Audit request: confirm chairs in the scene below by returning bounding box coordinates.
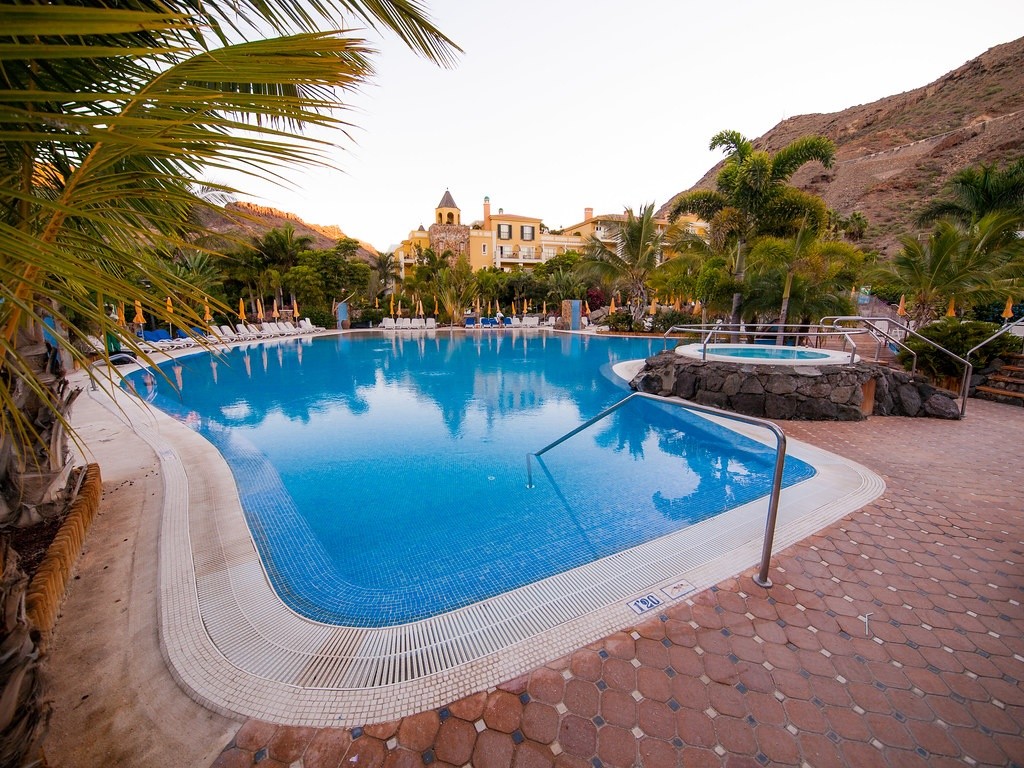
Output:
[220,325,244,343]
[209,326,231,344]
[176,329,198,348]
[236,324,257,341]
[873,321,919,338]
[276,322,296,337]
[401,318,410,329]
[299,320,316,333]
[306,318,327,333]
[384,319,395,329]
[284,322,307,335]
[136,331,170,351]
[247,324,270,340]
[269,323,287,338]
[378,318,389,328]
[393,318,403,329]
[260,322,281,339]
[410,317,588,330]
[192,327,217,346]
[152,329,184,350]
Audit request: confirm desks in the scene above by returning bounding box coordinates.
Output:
[892,330,905,337]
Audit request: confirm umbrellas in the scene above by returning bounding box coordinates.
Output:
[239,298,246,324]
[292,298,300,323]
[1001,293,1014,330]
[361,292,550,323]
[849,286,855,302]
[165,296,175,339]
[132,298,147,341]
[202,296,212,336]
[585,301,592,325]
[256,298,264,322]
[692,299,702,316]
[897,293,906,324]
[611,297,615,313]
[945,294,956,317]
[651,298,657,315]
[617,292,621,303]
[655,287,693,312]
[272,299,280,322]
[112,302,127,338]
[609,305,612,315]
